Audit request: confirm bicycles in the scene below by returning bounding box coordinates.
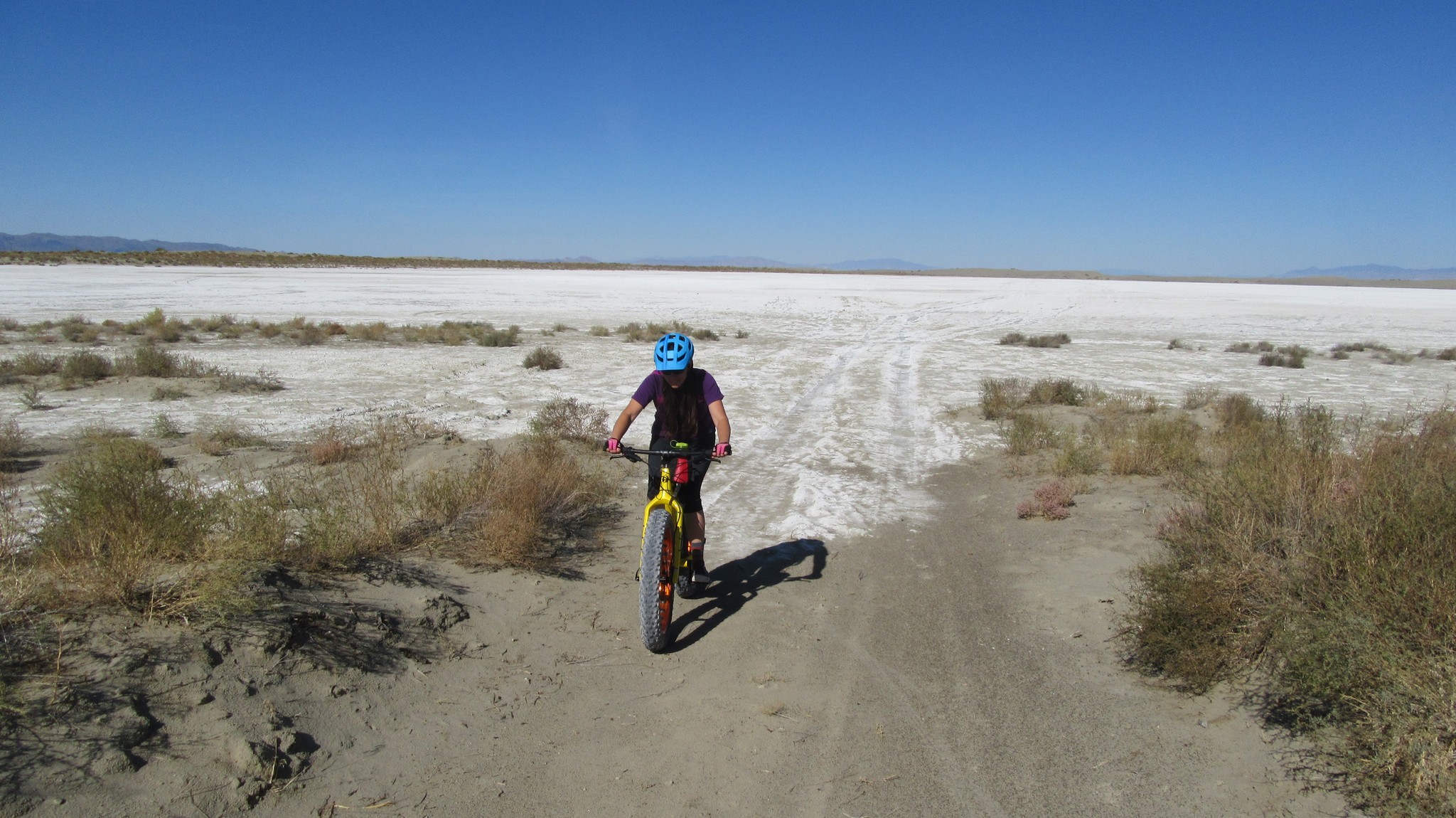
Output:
[602,444,733,654]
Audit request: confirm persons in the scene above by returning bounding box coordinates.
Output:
[603,334,730,584]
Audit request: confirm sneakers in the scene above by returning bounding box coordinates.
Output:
[687,548,710,583]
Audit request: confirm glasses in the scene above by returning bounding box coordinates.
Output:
[662,367,686,375]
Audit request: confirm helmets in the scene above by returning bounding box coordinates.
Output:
[654,333,694,370]
